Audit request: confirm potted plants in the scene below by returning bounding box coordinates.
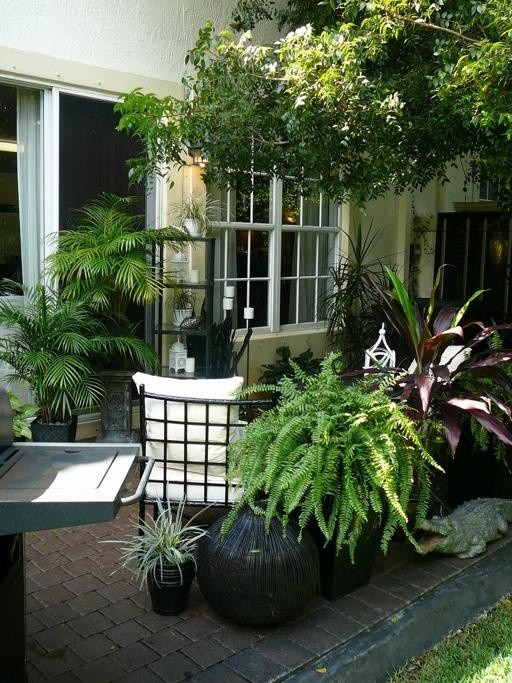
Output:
[44,189,197,443]
[164,275,201,328]
[167,184,223,238]
[0,277,160,442]
[342,261,512,540]
[218,342,446,603]
[97,488,217,615]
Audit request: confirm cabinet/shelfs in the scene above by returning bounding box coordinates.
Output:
[151,234,216,379]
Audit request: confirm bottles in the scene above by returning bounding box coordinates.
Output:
[169,334,188,377]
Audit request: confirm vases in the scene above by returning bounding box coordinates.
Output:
[452,202,479,211]
[489,232,508,273]
